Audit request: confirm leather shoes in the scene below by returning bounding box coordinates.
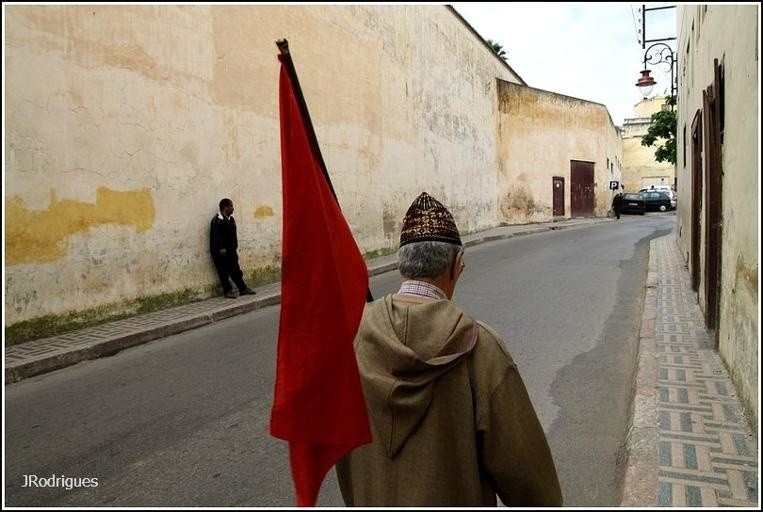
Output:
[240,288,256,295]
[224,292,237,299]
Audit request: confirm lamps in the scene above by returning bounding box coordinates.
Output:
[634,42,679,97]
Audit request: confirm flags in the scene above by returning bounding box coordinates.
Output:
[267,57,374,506]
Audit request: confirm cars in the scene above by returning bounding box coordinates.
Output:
[621,186,677,215]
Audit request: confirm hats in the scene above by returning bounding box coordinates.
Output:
[398,192,462,246]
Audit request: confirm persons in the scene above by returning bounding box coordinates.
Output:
[331,188,564,506]
[611,193,622,219]
[209,196,256,298]
